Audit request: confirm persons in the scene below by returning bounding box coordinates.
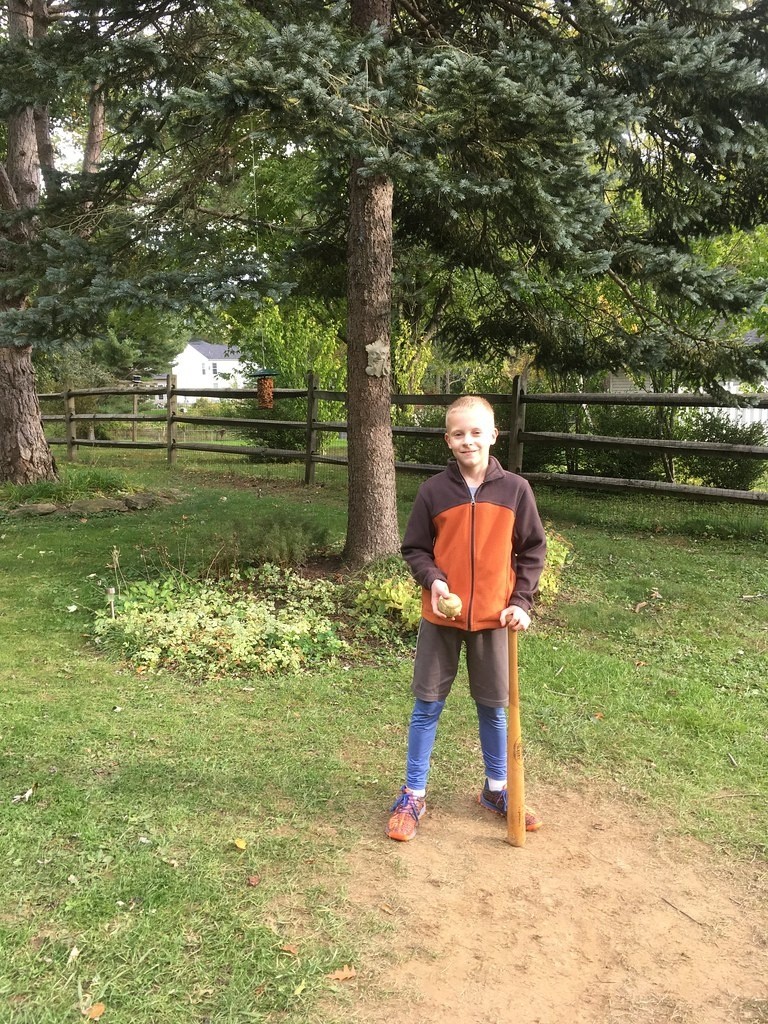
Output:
[387,395,549,842]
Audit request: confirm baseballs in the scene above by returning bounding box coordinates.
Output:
[437,592,462,618]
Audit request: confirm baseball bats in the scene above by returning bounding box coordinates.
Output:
[505,615,527,849]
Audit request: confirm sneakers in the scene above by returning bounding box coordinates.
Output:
[478,778,543,831]
[386,788,426,840]
